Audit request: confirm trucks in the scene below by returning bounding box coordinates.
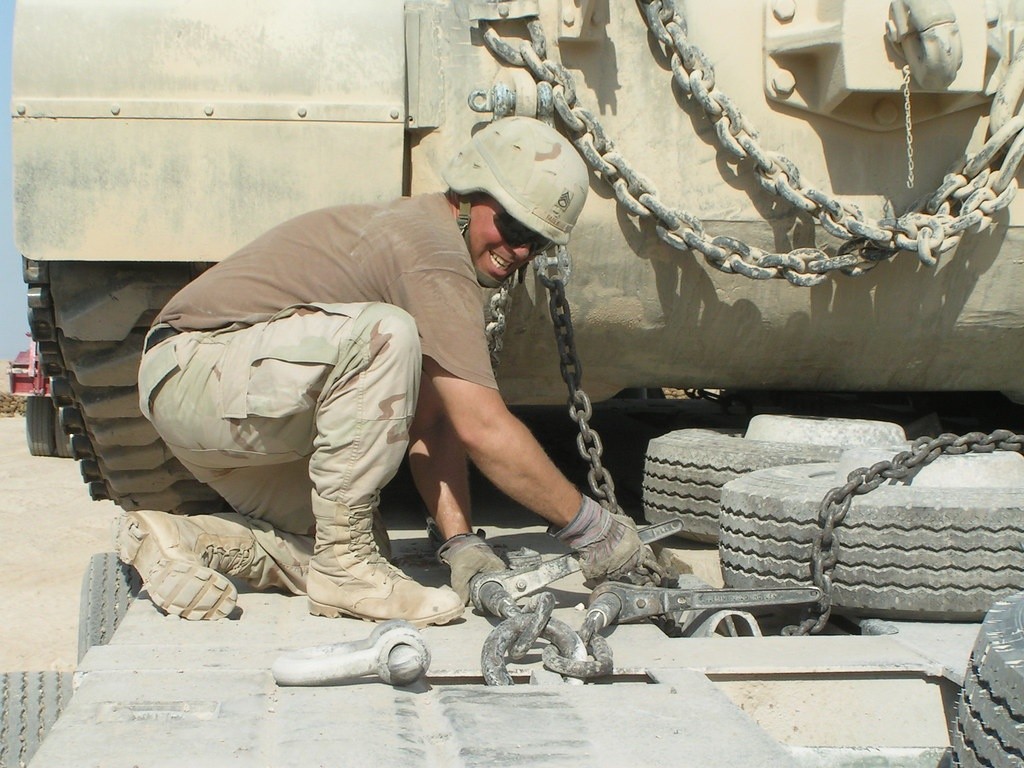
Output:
[6,330,75,458]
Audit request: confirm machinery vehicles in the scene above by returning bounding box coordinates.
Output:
[8,3,1024,517]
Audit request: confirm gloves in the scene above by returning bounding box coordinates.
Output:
[435,529,506,605]
[546,492,646,587]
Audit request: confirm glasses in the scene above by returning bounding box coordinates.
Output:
[494,211,554,260]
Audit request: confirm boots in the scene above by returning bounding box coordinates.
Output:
[110,513,255,620]
[307,487,465,629]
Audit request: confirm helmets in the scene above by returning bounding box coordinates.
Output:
[441,116,589,244]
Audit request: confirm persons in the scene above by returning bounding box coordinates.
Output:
[114,117,645,627]
